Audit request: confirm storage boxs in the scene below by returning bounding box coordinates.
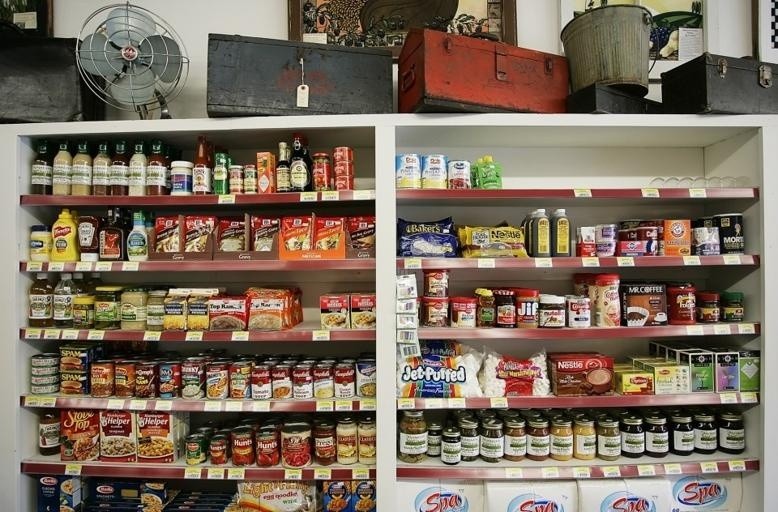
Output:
[398,28,568,114]
[661,52,778,114]
[207,33,393,116]
[567,83,661,114]
[1,36,104,122]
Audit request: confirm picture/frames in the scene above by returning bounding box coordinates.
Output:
[1,0,54,38]
[752,0,778,63]
[287,0,518,63]
[601,1,704,83]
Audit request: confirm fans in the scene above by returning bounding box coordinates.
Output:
[75,1,189,120]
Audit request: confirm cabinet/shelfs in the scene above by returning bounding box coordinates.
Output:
[0,113,376,512]
[395,115,764,511]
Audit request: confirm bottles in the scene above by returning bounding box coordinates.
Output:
[684,405,702,413]
[423,418,444,457]
[644,414,670,458]
[30,137,53,195]
[502,417,527,461]
[128,139,147,196]
[289,131,311,192]
[617,229,630,241]
[73,273,90,298]
[620,414,645,458]
[398,410,429,463]
[473,288,481,298]
[336,415,359,465]
[87,273,105,295]
[696,290,721,323]
[585,409,608,421]
[476,409,497,425]
[396,153,422,189]
[717,411,746,454]
[564,409,582,417]
[550,417,574,461]
[73,295,97,329]
[71,138,93,195]
[452,410,475,426]
[636,226,659,256]
[692,411,718,455]
[38,408,61,457]
[77,215,103,261]
[108,138,129,196]
[586,273,596,326]
[357,416,376,465]
[440,426,462,465]
[28,273,54,327]
[311,152,332,191]
[120,287,148,331]
[274,141,290,193]
[526,417,550,461]
[498,410,520,421]
[115,206,132,231]
[627,228,637,242]
[707,406,727,413]
[480,418,505,463]
[227,155,236,170]
[123,207,134,221]
[514,288,540,329]
[619,218,645,230]
[667,281,697,325]
[669,413,695,456]
[698,216,715,227]
[244,164,257,195]
[714,213,745,255]
[539,294,556,298]
[572,417,597,460]
[720,291,745,322]
[520,411,541,419]
[476,288,487,307]
[419,296,451,328]
[29,225,52,262]
[689,220,699,228]
[552,208,571,258]
[228,165,244,195]
[93,285,124,330]
[531,208,552,258]
[638,407,662,417]
[457,417,480,462]
[70,209,80,225]
[311,420,337,466]
[449,296,479,328]
[97,206,129,261]
[422,153,449,190]
[145,209,155,230]
[192,135,212,196]
[127,206,149,261]
[573,272,588,296]
[214,144,229,195]
[494,289,516,328]
[146,290,168,331]
[52,138,73,195]
[53,273,78,328]
[145,137,168,195]
[447,160,471,191]
[302,138,312,164]
[544,410,564,420]
[50,208,80,261]
[171,160,194,197]
[477,290,497,329]
[609,408,629,421]
[595,273,621,329]
[92,139,111,196]
[422,269,450,298]
[638,219,658,227]
[661,406,681,416]
[539,296,566,327]
[286,146,292,164]
[520,213,532,235]
[596,417,622,461]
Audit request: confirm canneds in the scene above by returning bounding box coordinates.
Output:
[299,359,318,364]
[153,356,173,360]
[359,351,376,357]
[251,364,273,401]
[292,364,314,399]
[271,364,293,400]
[335,362,355,369]
[194,425,214,456]
[157,359,183,398]
[209,347,227,355]
[237,418,260,431]
[312,364,335,399]
[236,357,255,361]
[205,420,222,434]
[135,361,159,400]
[134,355,153,360]
[202,355,215,366]
[199,350,217,357]
[281,359,299,365]
[251,353,272,359]
[283,416,312,426]
[255,426,279,468]
[288,356,300,360]
[114,360,139,399]
[274,353,290,357]
[231,353,249,359]
[185,432,208,465]
[319,359,337,364]
[212,356,234,362]
[174,356,187,361]
[334,362,356,398]
[292,354,303,357]
[219,428,235,458]
[59,342,97,396]
[181,356,206,400]
[230,425,255,467]
[227,362,252,398]
[111,352,130,358]
[259,360,280,366]
[339,356,356,364]
[303,355,321,362]
[90,358,115,398]
[281,422,313,469]
[355,357,376,398]
[206,361,229,401]
[30,352,60,395]
[332,145,356,191]
[268,356,284,361]
[322,355,339,360]
[209,433,229,464]
[258,417,283,430]
[361,352,376,358]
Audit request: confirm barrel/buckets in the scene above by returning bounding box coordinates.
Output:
[560,3,659,97]
